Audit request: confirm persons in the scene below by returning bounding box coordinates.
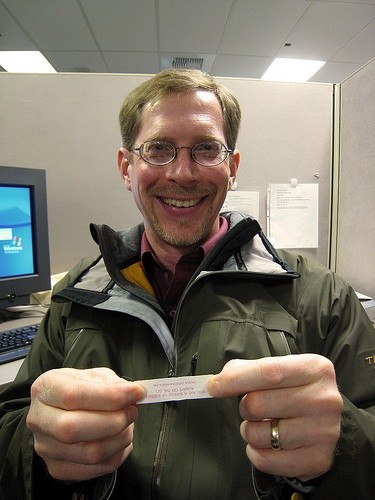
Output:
[0,68,375,500]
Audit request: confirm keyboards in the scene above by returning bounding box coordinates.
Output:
[0,322,41,364]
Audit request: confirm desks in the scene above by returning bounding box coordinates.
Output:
[0,305,45,393]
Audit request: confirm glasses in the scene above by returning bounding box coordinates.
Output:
[132,139,233,168]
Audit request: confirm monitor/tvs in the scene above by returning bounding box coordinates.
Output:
[0,166,51,309]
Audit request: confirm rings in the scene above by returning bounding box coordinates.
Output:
[270,418,281,451]
